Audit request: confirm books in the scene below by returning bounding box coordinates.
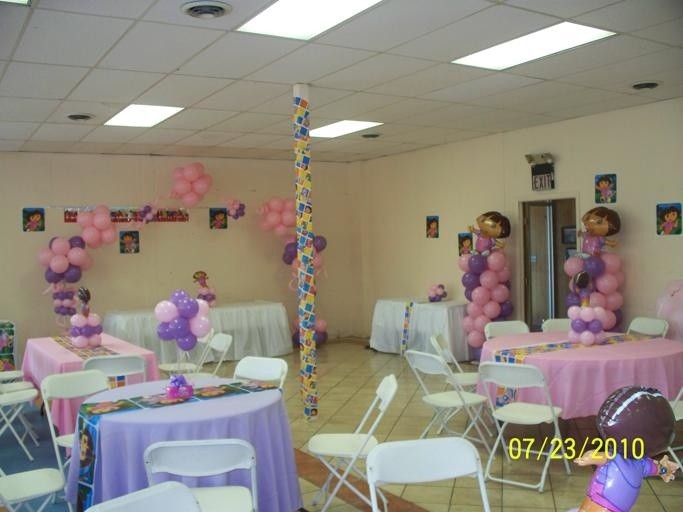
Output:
[312,235,326,269]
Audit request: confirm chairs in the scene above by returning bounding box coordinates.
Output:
[367,438,490,509]
[3,331,290,512]
[405,318,683,490]
[301,373,401,511]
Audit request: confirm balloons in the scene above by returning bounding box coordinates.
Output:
[167,163,213,210]
[257,197,298,291]
[315,318,328,346]
[127,200,160,226]
[38,206,117,348]
[292,318,299,348]
[226,199,246,221]
[428,283,447,302]
[563,207,625,345]
[457,212,514,361]
[573,384,679,512]
[155,271,217,351]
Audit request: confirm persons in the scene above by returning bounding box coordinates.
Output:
[660,206,680,233]
[428,220,438,236]
[459,237,473,256]
[73,382,280,511]
[24,211,41,229]
[211,210,225,227]
[595,178,614,202]
[120,233,136,253]
